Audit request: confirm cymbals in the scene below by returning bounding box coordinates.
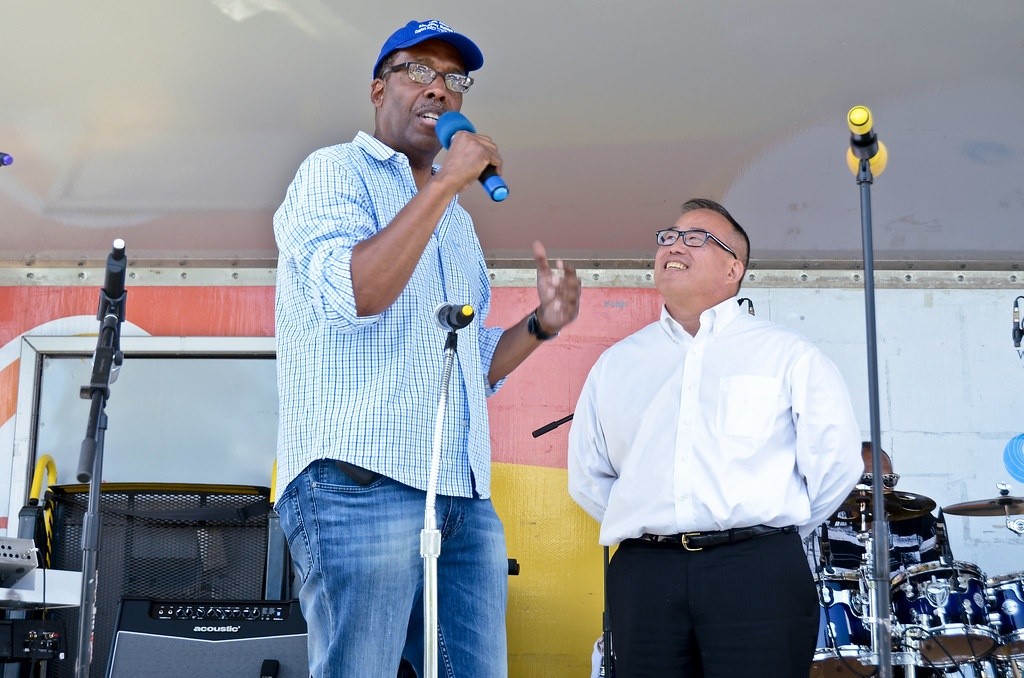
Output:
[942,495,1024,517]
[829,488,938,523]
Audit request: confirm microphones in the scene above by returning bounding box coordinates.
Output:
[433,110,510,202]
[433,301,475,330]
[260,659,279,678]
[1013,300,1021,347]
[91,238,126,384]
[846,106,888,178]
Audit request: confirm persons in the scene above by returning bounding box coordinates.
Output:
[843,441,895,529]
[566,197,864,678]
[272,18,581,678]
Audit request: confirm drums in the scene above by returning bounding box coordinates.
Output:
[890,559,999,665]
[984,571,1024,660]
[809,566,883,678]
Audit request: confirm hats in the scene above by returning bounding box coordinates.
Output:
[373,19,484,81]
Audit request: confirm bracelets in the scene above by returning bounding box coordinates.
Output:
[527,305,561,340]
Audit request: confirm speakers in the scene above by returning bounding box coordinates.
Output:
[104,597,312,678]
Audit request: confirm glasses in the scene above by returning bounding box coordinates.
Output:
[860,472,901,489]
[656,230,737,259]
[380,62,474,93]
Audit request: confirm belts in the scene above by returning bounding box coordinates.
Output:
[620,523,799,552]
